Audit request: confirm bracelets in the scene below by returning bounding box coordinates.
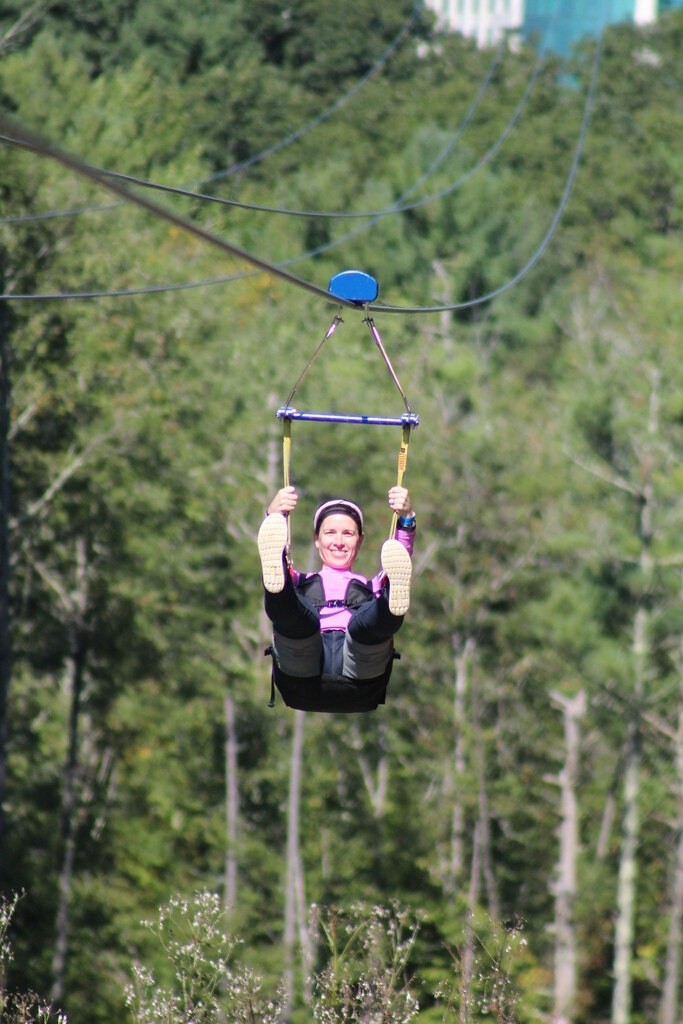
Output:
[399,510,416,526]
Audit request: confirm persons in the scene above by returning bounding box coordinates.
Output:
[256,485,417,714]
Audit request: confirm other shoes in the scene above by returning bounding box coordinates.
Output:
[381,539,413,616]
[257,512,288,594]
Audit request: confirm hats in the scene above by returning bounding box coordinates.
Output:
[314,500,364,536]
